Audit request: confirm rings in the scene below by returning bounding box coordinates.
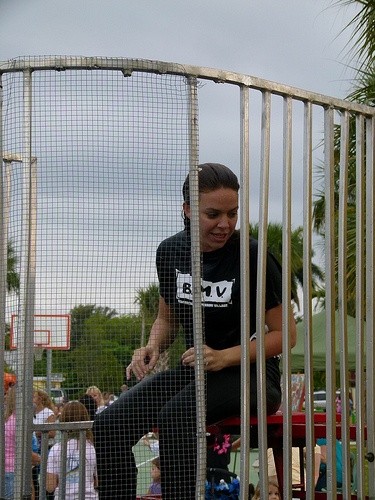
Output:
[203,358,209,366]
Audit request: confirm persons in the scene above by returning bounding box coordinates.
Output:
[3,372,65,500]
[141,430,161,496]
[79,383,130,422]
[335,395,353,416]
[205,432,354,492]
[45,401,99,500]
[93,163,297,500]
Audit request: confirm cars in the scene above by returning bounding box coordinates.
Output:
[50,389,68,405]
[303,391,353,410]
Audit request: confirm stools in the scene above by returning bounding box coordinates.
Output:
[207,411,369,500]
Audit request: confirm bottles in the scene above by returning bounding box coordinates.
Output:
[250,325,269,343]
